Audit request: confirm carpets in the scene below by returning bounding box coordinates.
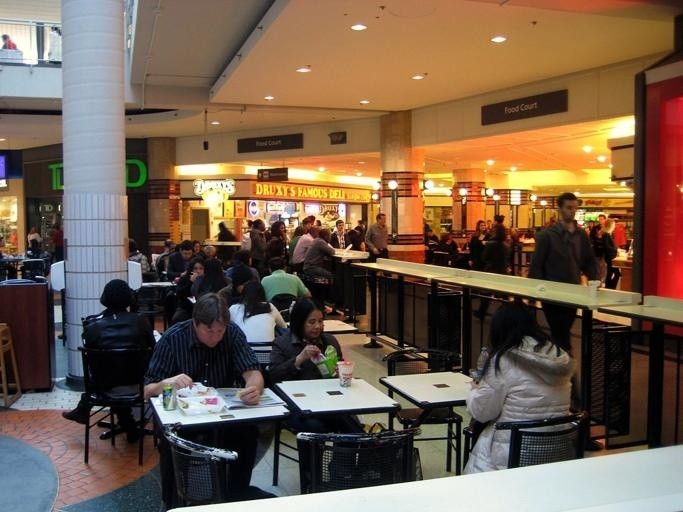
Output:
[0,432,59,512]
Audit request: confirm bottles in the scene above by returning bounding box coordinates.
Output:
[478,348,488,375]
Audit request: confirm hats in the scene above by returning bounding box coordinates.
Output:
[233,261,252,285]
[100,280,134,307]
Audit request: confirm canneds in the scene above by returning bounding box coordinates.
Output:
[162,385,176,410]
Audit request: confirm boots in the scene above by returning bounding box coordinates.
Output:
[63,393,92,423]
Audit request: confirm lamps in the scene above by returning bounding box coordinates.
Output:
[203,107,210,152]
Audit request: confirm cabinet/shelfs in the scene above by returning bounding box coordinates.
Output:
[0,277,57,392]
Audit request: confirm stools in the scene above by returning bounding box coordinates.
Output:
[1,321,23,409]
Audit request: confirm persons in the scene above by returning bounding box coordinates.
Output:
[214,208,389,309]
[527,191,628,356]
[457,301,570,473]
[421,215,520,280]
[148,293,364,509]
[48,26,62,65]
[59,242,312,433]
[2,34,16,50]
[0,237,17,279]
[27,223,63,266]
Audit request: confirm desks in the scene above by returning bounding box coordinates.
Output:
[460,269,642,457]
[594,293,682,450]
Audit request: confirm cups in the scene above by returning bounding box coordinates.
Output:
[337,361,354,387]
[588,279,600,298]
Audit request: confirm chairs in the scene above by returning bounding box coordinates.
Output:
[381,347,464,476]
[246,340,274,385]
[295,423,424,495]
[160,424,279,508]
[463,424,484,469]
[78,344,159,466]
[273,420,335,488]
[78,246,596,339]
[492,410,594,470]
